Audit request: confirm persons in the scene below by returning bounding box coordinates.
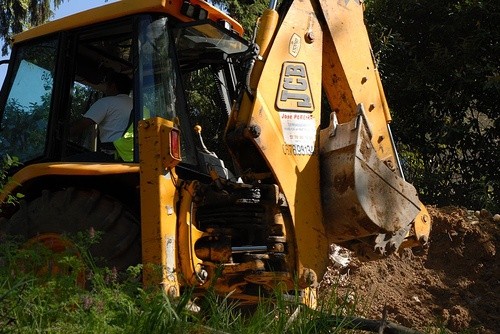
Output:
[63,72,133,162]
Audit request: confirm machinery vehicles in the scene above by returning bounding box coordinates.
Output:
[0,0,430,317]
